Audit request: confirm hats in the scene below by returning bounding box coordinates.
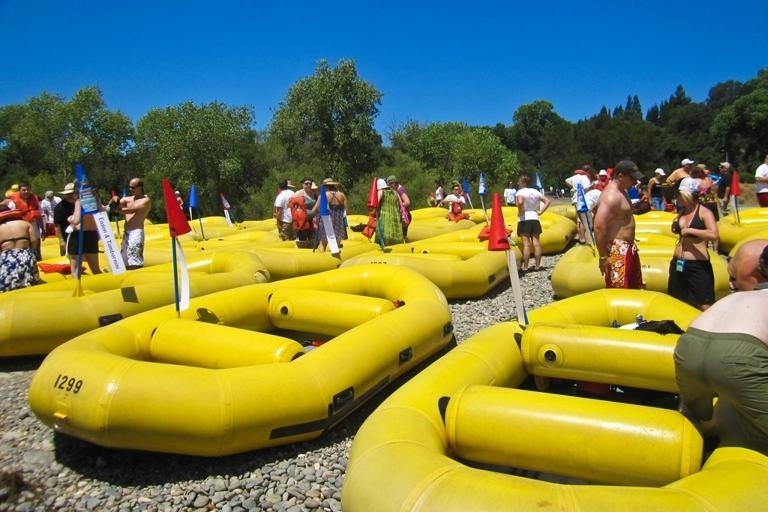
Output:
[663,176,701,196]
[612,159,646,180]
[323,177,340,185]
[720,161,730,169]
[597,169,608,176]
[376,178,391,190]
[681,158,695,167]
[300,177,319,190]
[276,179,296,188]
[44,190,54,201]
[57,182,75,195]
[4,183,21,199]
[654,167,666,176]
[386,175,398,185]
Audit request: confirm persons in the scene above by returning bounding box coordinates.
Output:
[430,175,564,273]
[1,177,184,290]
[274,175,413,250]
[563,157,768,452]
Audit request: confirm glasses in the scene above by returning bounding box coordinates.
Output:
[128,185,141,189]
[303,183,312,185]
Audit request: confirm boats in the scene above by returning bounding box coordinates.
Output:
[340,285,767,512]
[28,262,455,458]
